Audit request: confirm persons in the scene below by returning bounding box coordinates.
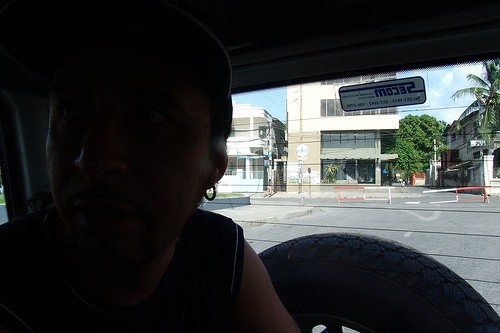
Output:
[1,3,304,332]
